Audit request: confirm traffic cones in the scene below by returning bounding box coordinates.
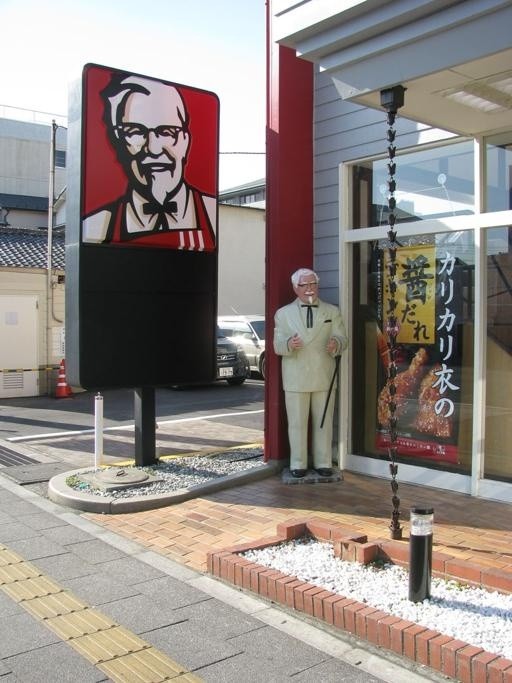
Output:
[54,358,73,399]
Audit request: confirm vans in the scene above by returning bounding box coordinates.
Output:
[217,314,265,380]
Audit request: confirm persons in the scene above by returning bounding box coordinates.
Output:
[273,267,348,479]
[79,74,218,253]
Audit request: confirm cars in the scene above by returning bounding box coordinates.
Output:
[217,325,251,384]
